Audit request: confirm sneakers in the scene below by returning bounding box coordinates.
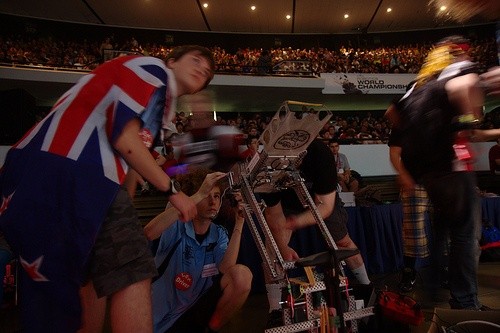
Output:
[399,267,416,292]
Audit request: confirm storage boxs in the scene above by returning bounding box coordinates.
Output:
[428,307,500,333]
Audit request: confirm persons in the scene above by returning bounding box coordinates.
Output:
[385,36,500,311]
[0,45,214,333]
[259,137,372,325]
[1,47,499,197]
[77,167,157,333]
[144,167,252,333]
[387,79,499,293]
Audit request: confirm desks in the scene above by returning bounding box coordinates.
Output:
[153,196,500,286]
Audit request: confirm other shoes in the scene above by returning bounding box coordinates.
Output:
[361,281,374,304]
[449,298,491,311]
[269,309,283,327]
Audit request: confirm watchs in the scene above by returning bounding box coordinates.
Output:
[162,179,180,197]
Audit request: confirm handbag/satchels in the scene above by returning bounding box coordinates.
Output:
[426,309,500,333]
